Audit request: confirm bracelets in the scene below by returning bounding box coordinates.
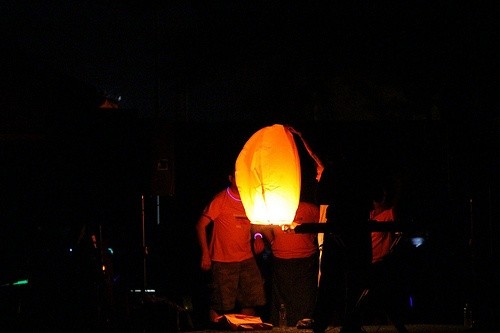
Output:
[254,232,262,240]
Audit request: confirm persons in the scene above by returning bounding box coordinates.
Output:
[260,124,325,327]
[197,162,267,316]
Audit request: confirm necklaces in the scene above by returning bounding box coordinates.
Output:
[226,186,243,202]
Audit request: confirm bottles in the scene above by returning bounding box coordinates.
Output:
[279,304,287,328]
